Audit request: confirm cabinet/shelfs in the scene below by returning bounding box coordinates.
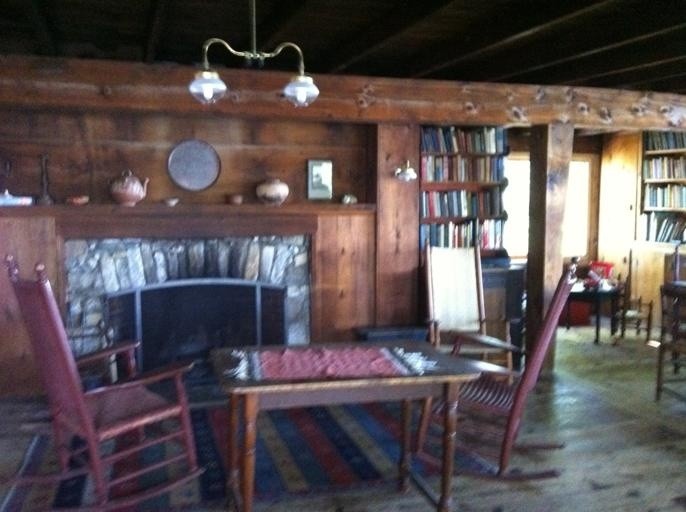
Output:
[0,205,374,395]
[420,125,526,371]
[598,131,686,333]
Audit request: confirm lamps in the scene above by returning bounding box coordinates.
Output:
[188,0,320,109]
[391,159,417,183]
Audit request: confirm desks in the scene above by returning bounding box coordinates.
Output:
[571,279,621,334]
[214,341,480,512]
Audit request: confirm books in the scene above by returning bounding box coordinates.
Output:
[640,212,686,245]
[415,187,506,220]
[644,129,686,149]
[418,222,505,254]
[641,183,686,208]
[641,158,685,180]
[418,156,507,185]
[417,126,510,153]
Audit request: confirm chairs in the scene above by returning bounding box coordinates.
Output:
[423,239,517,389]
[4,254,206,512]
[419,267,578,476]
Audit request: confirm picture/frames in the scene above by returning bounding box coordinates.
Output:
[306,158,333,199]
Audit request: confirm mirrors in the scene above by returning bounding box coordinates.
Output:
[164,138,222,193]
